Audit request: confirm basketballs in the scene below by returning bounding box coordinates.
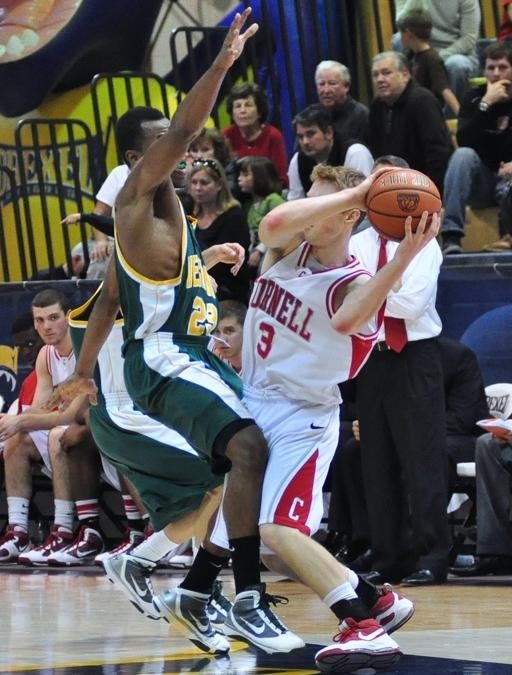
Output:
[366,169,442,242]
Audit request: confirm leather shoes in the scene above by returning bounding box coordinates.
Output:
[324,532,505,586]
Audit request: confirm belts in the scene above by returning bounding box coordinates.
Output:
[374,342,416,351]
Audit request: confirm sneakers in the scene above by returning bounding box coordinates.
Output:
[98,527,163,621]
[0,524,143,566]
[315,583,415,673]
[442,235,462,254]
[483,237,512,252]
[155,579,306,655]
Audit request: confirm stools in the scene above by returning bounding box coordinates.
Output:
[444,460,478,563]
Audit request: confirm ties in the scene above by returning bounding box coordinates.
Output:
[378,236,407,353]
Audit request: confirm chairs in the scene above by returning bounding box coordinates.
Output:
[27,469,126,556]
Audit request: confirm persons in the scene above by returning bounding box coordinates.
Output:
[219,80,290,188]
[0,103,511,587]
[314,59,371,132]
[65,240,236,637]
[41,5,308,663]
[240,161,443,673]
[389,0,482,99]
[359,50,455,196]
[438,41,511,255]
[395,8,462,117]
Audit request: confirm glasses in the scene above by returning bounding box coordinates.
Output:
[192,159,221,176]
